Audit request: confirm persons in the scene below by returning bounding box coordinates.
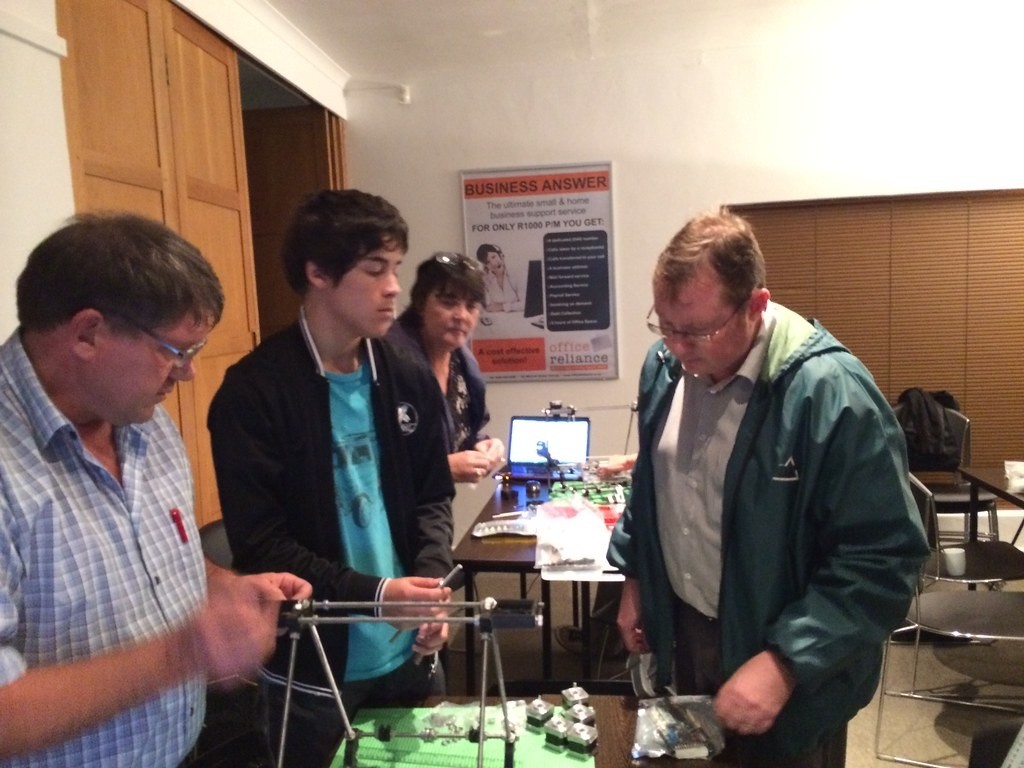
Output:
[0,213,314,767]
[395,250,505,484]
[205,188,455,767]
[477,242,528,311]
[606,215,932,768]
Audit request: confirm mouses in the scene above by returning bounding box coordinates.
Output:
[481,316,493,325]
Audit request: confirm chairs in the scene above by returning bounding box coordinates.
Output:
[875,387,1024,766]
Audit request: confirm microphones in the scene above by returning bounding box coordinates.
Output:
[498,258,504,268]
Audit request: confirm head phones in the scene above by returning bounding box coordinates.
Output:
[483,244,505,274]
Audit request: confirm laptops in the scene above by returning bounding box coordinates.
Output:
[493,416,590,480]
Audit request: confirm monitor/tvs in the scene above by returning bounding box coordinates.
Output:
[524,259,545,331]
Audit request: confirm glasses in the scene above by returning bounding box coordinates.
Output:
[645,283,758,346]
[97,306,208,369]
[432,249,483,272]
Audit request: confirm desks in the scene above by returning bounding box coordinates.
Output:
[958,467,1024,591]
[452,482,635,693]
[327,696,717,768]
